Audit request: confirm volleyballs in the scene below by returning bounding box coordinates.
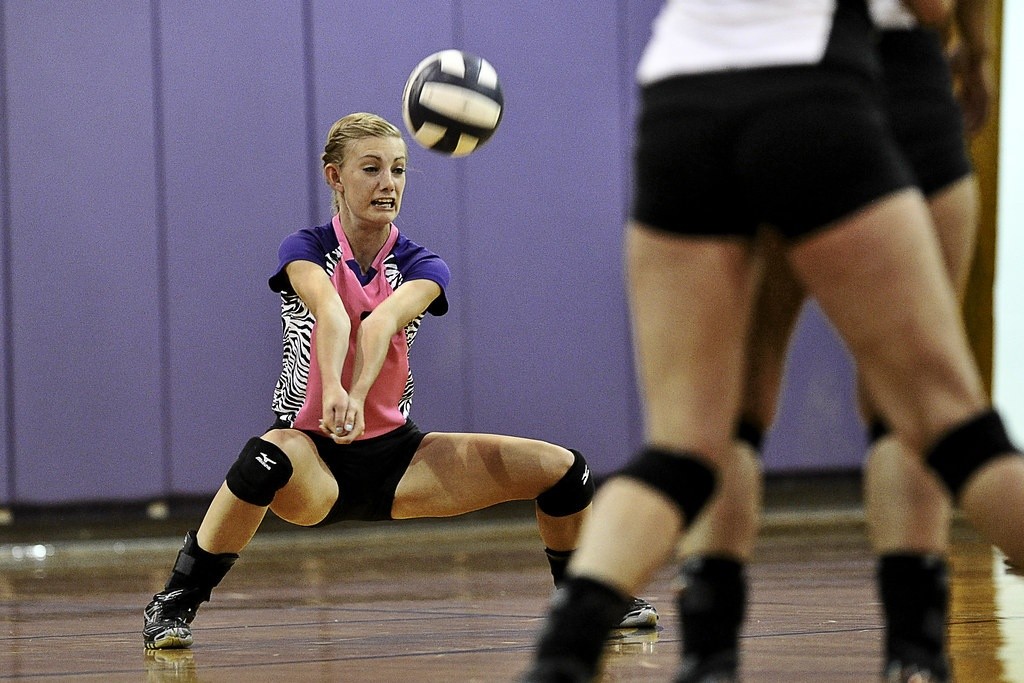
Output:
[400,48,504,157]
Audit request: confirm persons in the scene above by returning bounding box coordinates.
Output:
[141,113,660,649]
[518,0,1024,683]
[660,0,985,683]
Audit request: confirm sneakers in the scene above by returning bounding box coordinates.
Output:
[143,650,195,672]
[618,597,659,626]
[143,587,192,649]
[609,628,656,654]
